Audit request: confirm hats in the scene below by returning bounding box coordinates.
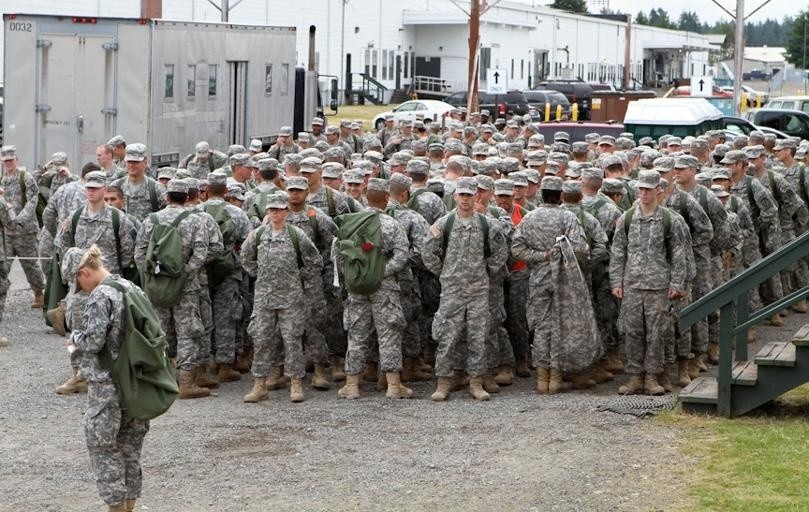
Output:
[183,178,199,189]
[352,122,361,130]
[177,169,192,178]
[445,138,461,146]
[325,147,345,157]
[341,121,353,128]
[430,122,440,129]
[602,179,628,195]
[450,106,479,119]
[481,110,489,117]
[298,131,310,142]
[228,145,246,154]
[507,142,522,152]
[562,180,582,192]
[364,150,384,164]
[596,153,611,167]
[369,139,382,148]
[52,152,68,171]
[658,134,674,143]
[494,179,515,196]
[506,120,518,129]
[466,158,472,167]
[385,115,394,122]
[258,158,278,170]
[451,122,464,133]
[502,157,524,173]
[715,143,730,156]
[428,144,444,152]
[682,135,694,145]
[512,115,522,122]
[85,170,107,187]
[690,137,711,152]
[285,154,304,166]
[541,176,562,191]
[156,167,177,179]
[314,140,329,149]
[640,149,662,167]
[197,180,207,192]
[227,186,245,201]
[407,160,429,174]
[449,155,467,168]
[324,127,339,135]
[471,159,481,175]
[251,152,271,168]
[464,126,479,135]
[660,150,669,154]
[392,172,413,202]
[207,173,227,185]
[352,161,373,174]
[355,121,363,126]
[572,141,589,153]
[368,178,391,194]
[249,140,263,152]
[704,131,719,139]
[639,146,652,150]
[403,120,412,126]
[719,150,746,164]
[603,155,623,169]
[414,156,431,166]
[527,124,539,132]
[265,194,289,211]
[107,135,125,150]
[0,145,16,160]
[195,141,208,158]
[413,121,423,128]
[581,167,603,178]
[508,171,528,187]
[613,151,628,161]
[312,117,324,126]
[794,150,806,160]
[481,124,494,133]
[798,139,809,153]
[525,150,548,166]
[475,175,494,190]
[489,146,498,155]
[473,143,489,155]
[386,153,411,166]
[522,113,532,124]
[712,168,732,180]
[632,147,644,152]
[299,157,322,173]
[493,132,504,141]
[710,184,730,198]
[521,168,541,183]
[549,152,569,163]
[695,172,711,184]
[545,160,560,174]
[599,135,615,147]
[712,131,726,139]
[554,131,570,140]
[286,176,309,190]
[479,161,500,175]
[750,130,764,143]
[639,137,656,145]
[585,133,600,143]
[553,142,571,152]
[279,126,292,137]
[167,180,189,194]
[416,114,424,120]
[745,144,765,158]
[299,148,325,162]
[675,155,699,169]
[667,137,682,146]
[788,136,801,146]
[638,170,661,189]
[427,179,444,193]
[486,157,503,169]
[528,134,544,147]
[772,139,796,150]
[279,168,287,181]
[61,247,85,295]
[321,162,345,178]
[670,152,683,157]
[564,161,592,178]
[411,140,426,152]
[734,135,748,149]
[343,171,364,183]
[616,132,636,147]
[230,154,250,165]
[124,143,146,162]
[456,177,478,194]
[764,133,777,142]
[653,157,674,172]
[231,183,247,194]
[494,118,506,125]
[428,135,443,143]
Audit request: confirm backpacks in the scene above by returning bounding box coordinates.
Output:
[95,278,180,421]
[334,211,386,294]
[142,210,193,306]
[20,170,48,227]
[204,203,235,289]
[250,186,279,219]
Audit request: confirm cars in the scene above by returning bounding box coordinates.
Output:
[756,125,799,140]
[373,97,457,130]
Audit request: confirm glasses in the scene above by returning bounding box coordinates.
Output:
[348,182,361,186]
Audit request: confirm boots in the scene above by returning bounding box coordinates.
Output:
[31,291,44,308]
[338,375,360,398]
[771,312,784,326]
[678,360,691,386]
[709,343,720,365]
[194,366,219,387]
[233,352,250,371]
[57,373,89,394]
[470,377,490,400]
[431,377,450,400]
[484,375,500,393]
[416,362,432,372]
[244,378,268,402]
[494,365,514,384]
[401,357,432,381]
[331,356,346,381]
[386,372,413,398]
[207,363,218,377]
[219,364,241,382]
[377,374,387,390]
[645,373,665,395]
[549,369,573,394]
[46,303,68,336]
[536,367,549,394]
[565,356,624,388]
[696,354,708,372]
[311,363,329,389]
[180,370,210,399]
[265,366,287,390]
[517,366,531,377]
[290,378,304,402]
[619,374,644,394]
[659,374,673,392]
[363,365,378,381]
[689,362,699,378]
[793,302,807,313]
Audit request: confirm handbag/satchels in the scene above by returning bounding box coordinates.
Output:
[43,246,66,327]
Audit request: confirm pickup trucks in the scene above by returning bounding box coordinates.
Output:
[744,70,772,80]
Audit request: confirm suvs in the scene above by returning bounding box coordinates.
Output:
[582,83,617,95]
[476,91,529,116]
[718,85,768,104]
[515,90,573,118]
[747,108,809,135]
[446,92,491,115]
[678,85,736,99]
[533,82,595,113]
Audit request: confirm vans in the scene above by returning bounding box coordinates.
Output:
[770,96,809,113]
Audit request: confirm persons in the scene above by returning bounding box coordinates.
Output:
[239,190,324,401]
[59,244,179,511]
[421,176,509,401]
[331,176,416,399]
[408,89,417,100]
[1,106,809,401]
[607,170,688,394]
